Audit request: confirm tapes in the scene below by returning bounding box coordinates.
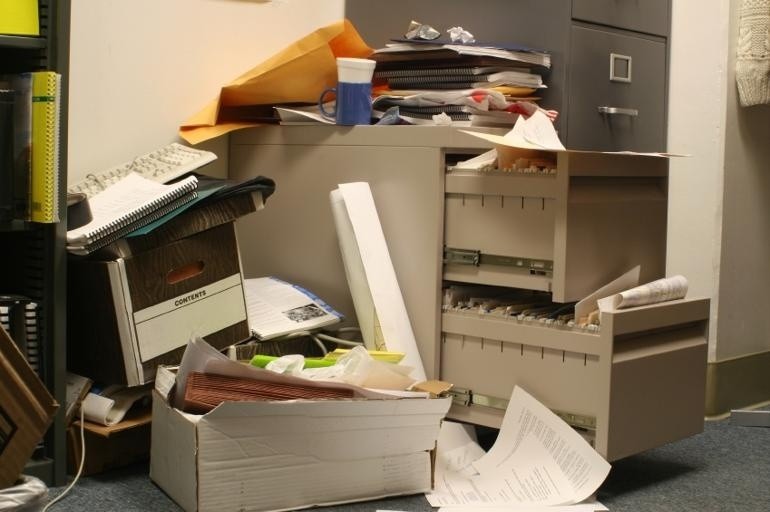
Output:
[67,192,93,231]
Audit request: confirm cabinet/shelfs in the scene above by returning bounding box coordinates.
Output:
[228,126,711,461]
[1,1,70,488]
[344,0,672,151]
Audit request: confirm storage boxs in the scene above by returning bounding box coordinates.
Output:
[150,349,453,511]
[68,219,252,387]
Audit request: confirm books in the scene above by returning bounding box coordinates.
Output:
[370,36,550,128]
[0,71,198,259]
[243,274,346,342]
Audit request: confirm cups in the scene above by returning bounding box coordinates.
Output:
[319,80,374,126]
[335,55,376,85]
